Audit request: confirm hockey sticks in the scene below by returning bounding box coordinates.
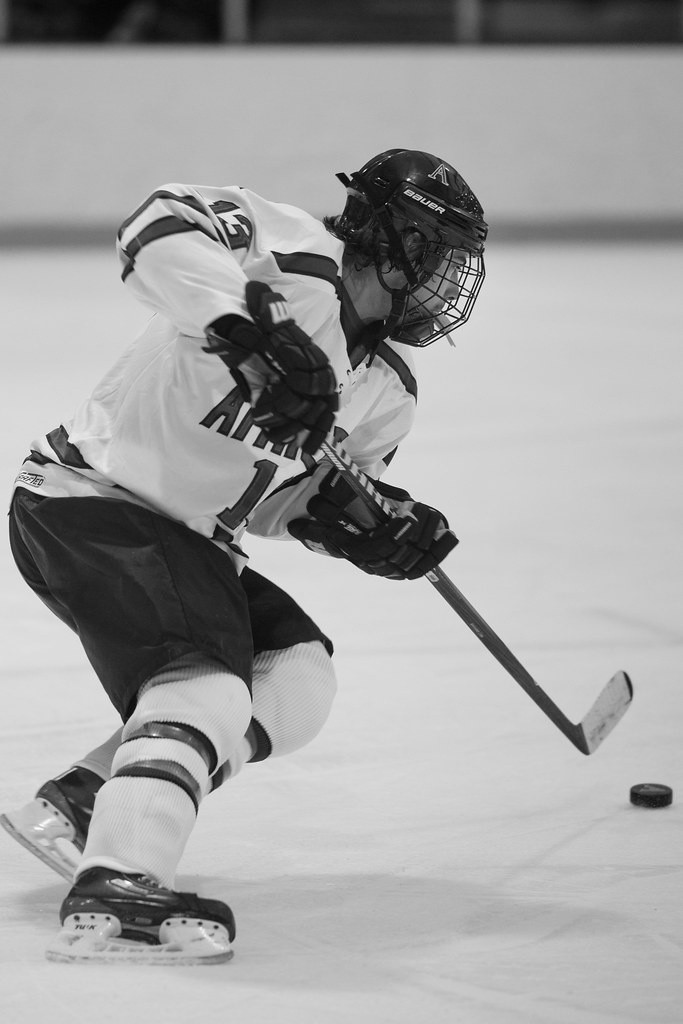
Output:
[266,372,633,756]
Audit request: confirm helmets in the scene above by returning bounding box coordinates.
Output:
[335,149,490,347]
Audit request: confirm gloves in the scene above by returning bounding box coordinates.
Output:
[287,467,459,581]
[201,280,339,454]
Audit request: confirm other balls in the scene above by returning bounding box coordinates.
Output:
[630,784,675,807]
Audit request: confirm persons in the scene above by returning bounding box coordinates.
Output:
[1,148,490,967]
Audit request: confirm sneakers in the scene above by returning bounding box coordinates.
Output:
[46,866,236,967]
[0,766,106,884]
